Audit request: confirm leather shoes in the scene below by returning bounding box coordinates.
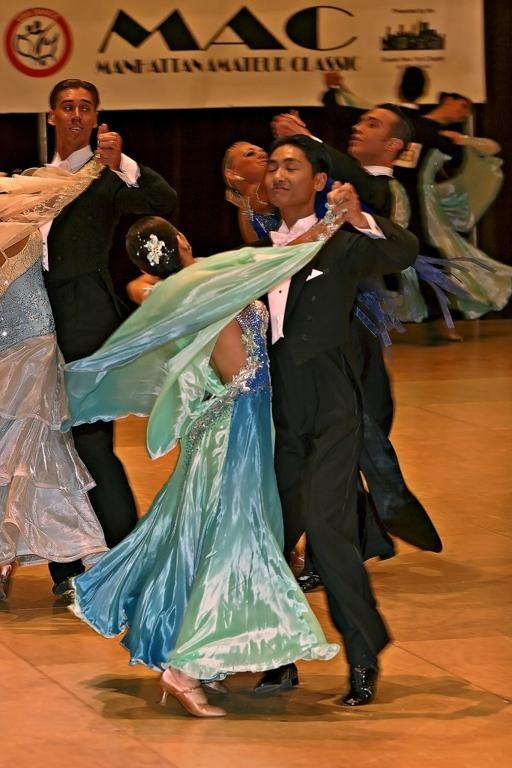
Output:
[254,663,299,693]
[344,665,377,705]
[296,570,322,592]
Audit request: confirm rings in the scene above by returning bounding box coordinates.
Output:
[94,153,102,159]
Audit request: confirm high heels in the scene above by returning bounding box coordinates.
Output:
[200,681,227,694]
[0,575,8,602]
[158,667,226,717]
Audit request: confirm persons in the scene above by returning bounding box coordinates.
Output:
[221,113,400,572]
[334,72,512,327]
[320,64,472,342]
[45,77,180,613]
[238,133,422,707]
[1,123,106,615]
[272,101,445,594]
[65,180,348,718]
